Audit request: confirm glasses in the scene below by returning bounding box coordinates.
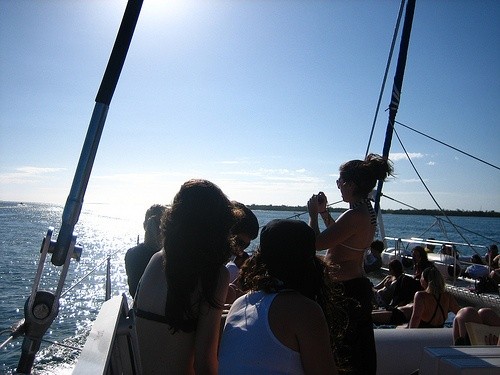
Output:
[236,239,250,250]
[145,220,162,234]
[337,180,345,188]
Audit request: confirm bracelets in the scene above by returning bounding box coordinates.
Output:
[320,211,327,214]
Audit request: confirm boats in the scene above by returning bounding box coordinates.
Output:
[366,187,500,310]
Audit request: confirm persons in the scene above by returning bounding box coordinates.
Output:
[124,153,500,375]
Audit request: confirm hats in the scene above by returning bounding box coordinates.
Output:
[260,219,316,262]
